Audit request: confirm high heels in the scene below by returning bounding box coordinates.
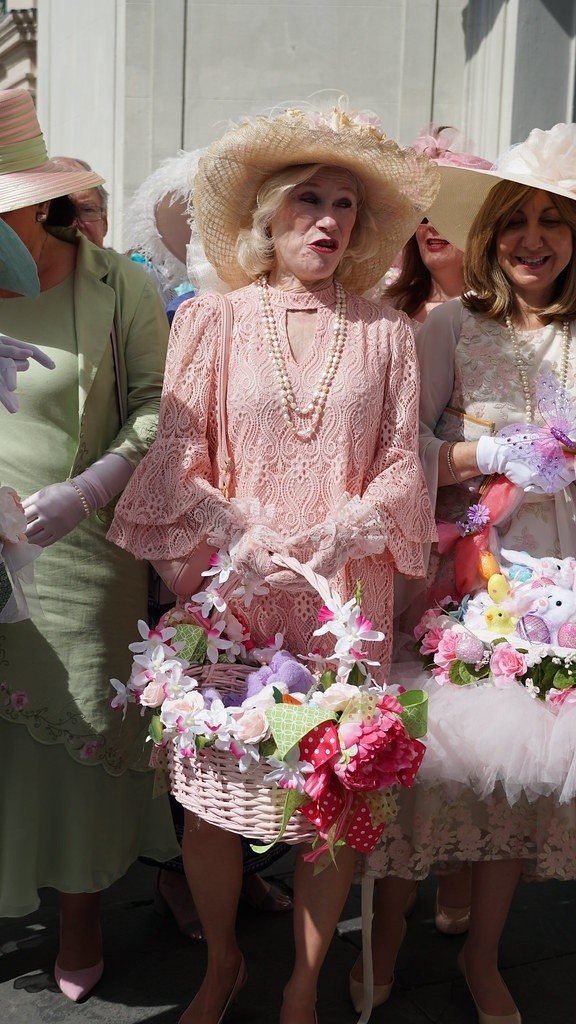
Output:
[436,878,470,937]
[351,913,406,1011]
[461,939,520,1023]
[153,865,208,947]
[313,1005,318,1024]
[405,876,418,916]
[183,950,247,1023]
[240,869,293,915]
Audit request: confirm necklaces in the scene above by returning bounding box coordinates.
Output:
[503,311,569,429]
[255,274,347,440]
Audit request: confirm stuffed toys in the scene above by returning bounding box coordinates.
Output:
[463,546,576,650]
[199,646,318,710]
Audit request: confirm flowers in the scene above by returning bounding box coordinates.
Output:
[416,549,576,802]
[109,548,428,873]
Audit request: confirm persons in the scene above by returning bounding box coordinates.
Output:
[365,131,501,932]
[121,138,293,945]
[98,102,444,1023]
[348,124,576,1022]
[0,89,169,1005]
[51,156,111,247]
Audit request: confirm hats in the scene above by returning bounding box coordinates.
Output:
[414,122,576,253]
[191,111,440,297]
[403,124,495,173]
[133,150,212,286]
[0,88,105,217]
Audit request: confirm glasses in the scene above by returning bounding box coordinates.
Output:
[76,202,104,223]
[419,216,429,225]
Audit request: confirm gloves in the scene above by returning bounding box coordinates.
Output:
[0,332,56,413]
[476,432,576,495]
[20,454,133,547]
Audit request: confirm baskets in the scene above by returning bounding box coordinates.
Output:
[125,553,390,843]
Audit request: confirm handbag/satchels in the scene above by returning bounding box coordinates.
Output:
[147,535,219,599]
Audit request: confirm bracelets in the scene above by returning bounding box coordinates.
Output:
[446,441,461,484]
[66,477,90,517]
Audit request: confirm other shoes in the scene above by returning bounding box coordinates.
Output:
[54,908,104,1002]
